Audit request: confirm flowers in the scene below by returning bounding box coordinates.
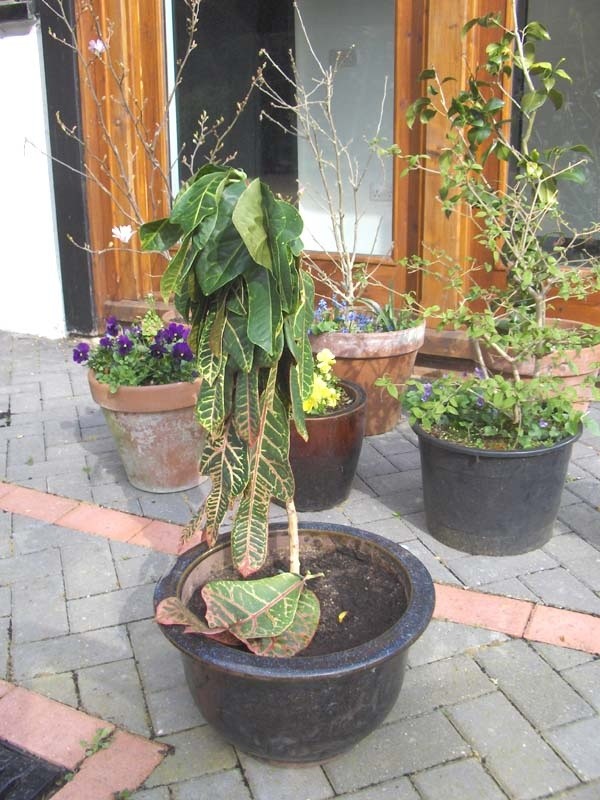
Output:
[302,348,341,415]
[72,290,194,394]
[35,0,259,269]
[406,368,583,450]
[311,293,396,339]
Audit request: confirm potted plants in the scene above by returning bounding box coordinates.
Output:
[86,0,599,768]
